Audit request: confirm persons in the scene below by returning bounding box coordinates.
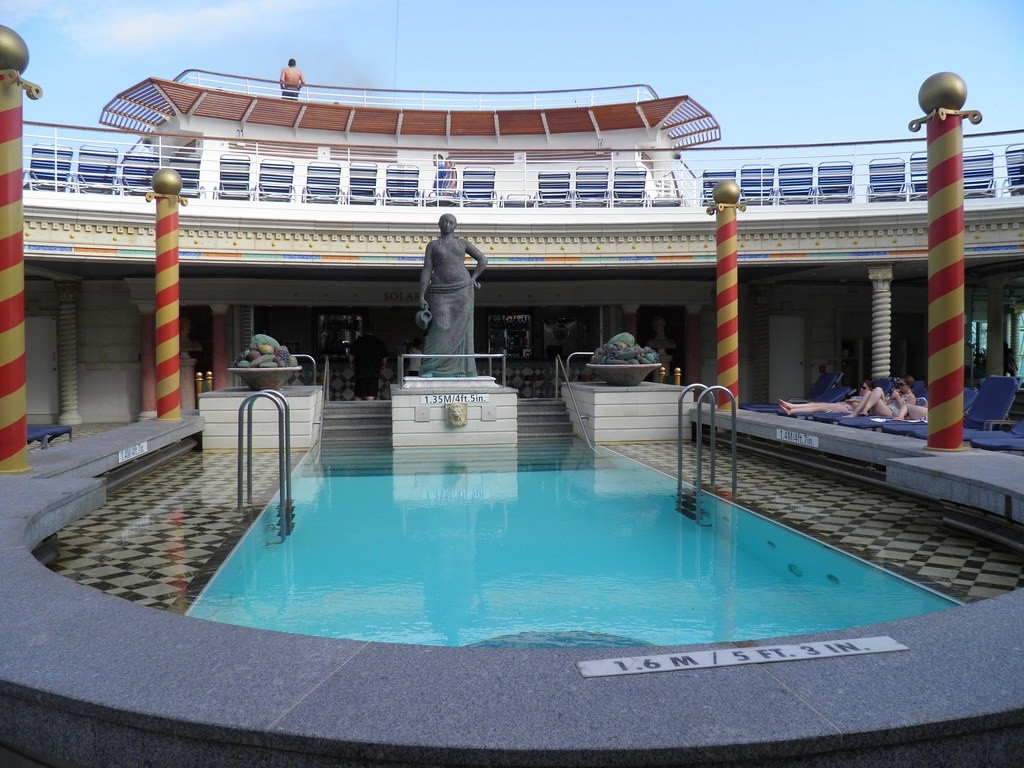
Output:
[418,212,489,377]
[648,317,676,347]
[406,338,424,376]
[1003,341,1018,377]
[777,375,929,422]
[431,153,457,196]
[179,317,203,351]
[347,321,388,402]
[280,58,305,101]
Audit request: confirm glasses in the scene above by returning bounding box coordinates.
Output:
[861,386,868,390]
[897,383,908,386]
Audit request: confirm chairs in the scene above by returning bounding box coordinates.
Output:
[16,138,1023,210]
[739,370,1024,455]
[27,425,72,451]
